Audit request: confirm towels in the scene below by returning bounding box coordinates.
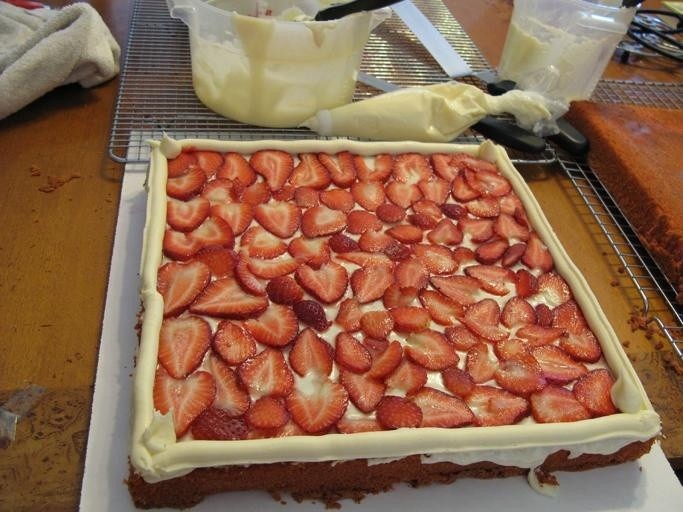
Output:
[0,4,123,127]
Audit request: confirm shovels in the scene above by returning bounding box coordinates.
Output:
[389,0,590,158]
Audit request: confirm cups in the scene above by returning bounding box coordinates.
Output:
[494,0,641,113]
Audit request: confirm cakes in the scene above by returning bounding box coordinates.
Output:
[127,129,662,510]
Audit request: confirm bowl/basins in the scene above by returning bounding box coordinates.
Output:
[165,0,392,130]
